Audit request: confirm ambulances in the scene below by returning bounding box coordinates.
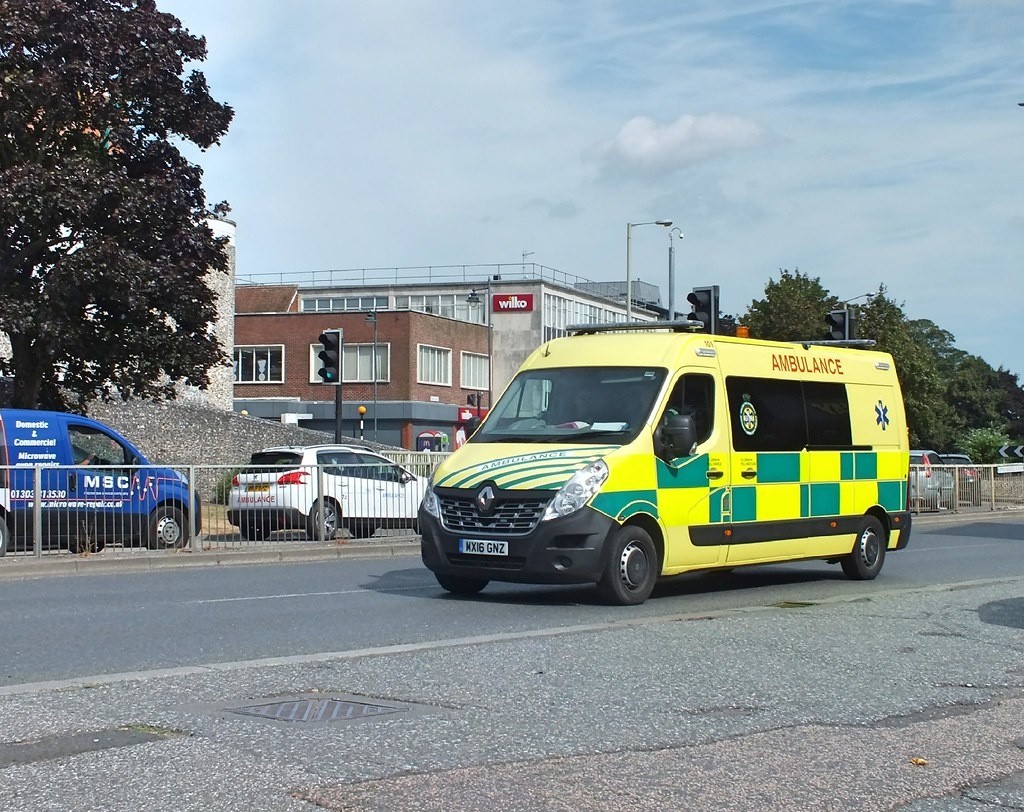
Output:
[418,320,911,606]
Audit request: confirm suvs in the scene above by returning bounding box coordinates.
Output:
[227,444,429,542]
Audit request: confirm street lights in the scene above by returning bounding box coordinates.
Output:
[843,293,876,309]
[668,227,684,333]
[358,405,366,440]
[363,310,377,442]
[627,222,673,333]
[465,277,490,410]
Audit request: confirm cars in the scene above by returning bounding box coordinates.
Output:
[910,450,981,512]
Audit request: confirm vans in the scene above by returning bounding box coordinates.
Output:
[0,409,201,557]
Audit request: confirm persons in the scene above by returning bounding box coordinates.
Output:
[539,387,597,425]
[637,386,679,430]
[74,452,94,465]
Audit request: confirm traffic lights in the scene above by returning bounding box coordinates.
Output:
[318,328,343,385]
[825,310,848,340]
[687,285,719,334]
